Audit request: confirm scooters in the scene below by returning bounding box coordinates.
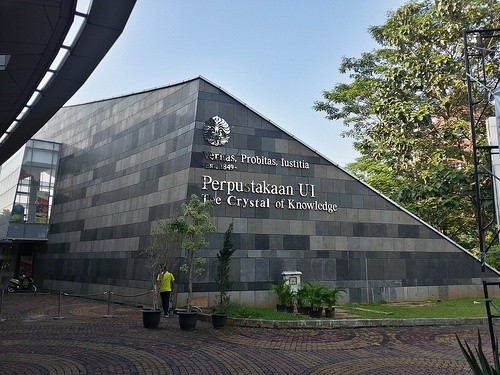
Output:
[5,274,37,293]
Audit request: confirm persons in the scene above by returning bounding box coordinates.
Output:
[155,266,173,319]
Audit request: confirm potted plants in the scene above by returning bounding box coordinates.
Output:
[267,278,348,318]
[211,222,238,328]
[138,207,185,329]
[170,193,219,331]
